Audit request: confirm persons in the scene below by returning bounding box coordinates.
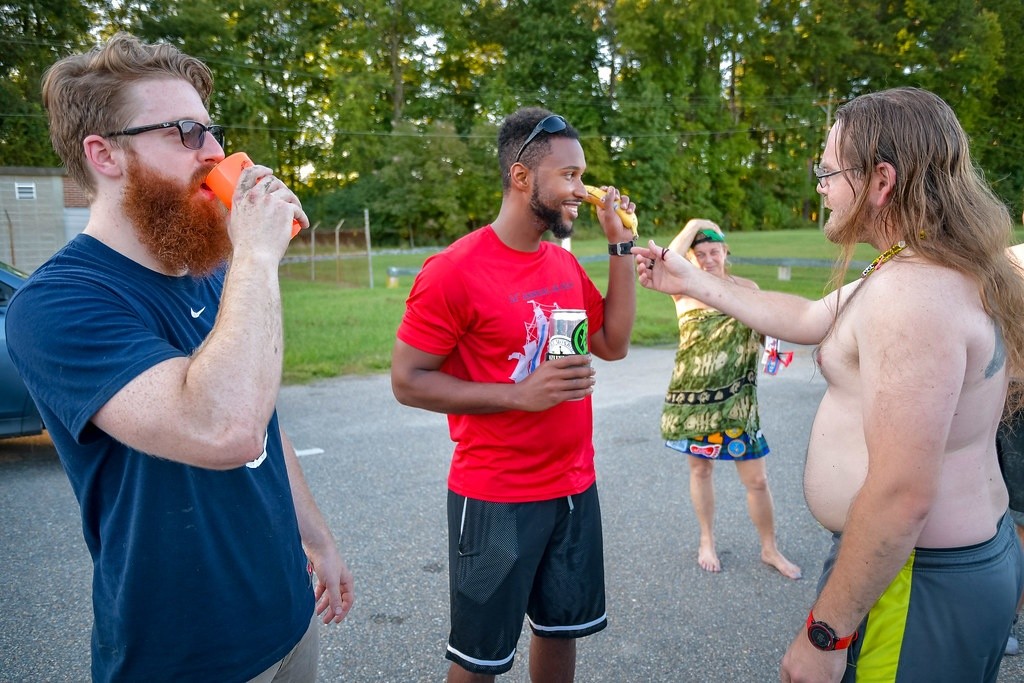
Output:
[995,211,1024,538]
[388,105,640,683]
[629,87,1022,683]
[5,32,356,681]
[659,219,805,580]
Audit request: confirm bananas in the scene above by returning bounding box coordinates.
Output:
[576,184,642,238]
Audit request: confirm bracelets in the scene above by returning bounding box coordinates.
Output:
[661,248,669,260]
[608,241,636,257]
[649,259,654,270]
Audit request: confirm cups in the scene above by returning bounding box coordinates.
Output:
[206,152,302,242]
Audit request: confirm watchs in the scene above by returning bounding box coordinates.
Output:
[807,612,856,651]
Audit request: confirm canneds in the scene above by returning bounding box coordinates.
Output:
[208,149,300,245]
[546,308,591,404]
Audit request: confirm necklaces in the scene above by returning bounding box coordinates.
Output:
[860,229,926,279]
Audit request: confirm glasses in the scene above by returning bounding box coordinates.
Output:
[509,116,568,174]
[101,118,228,153]
[816,164,868,188]
